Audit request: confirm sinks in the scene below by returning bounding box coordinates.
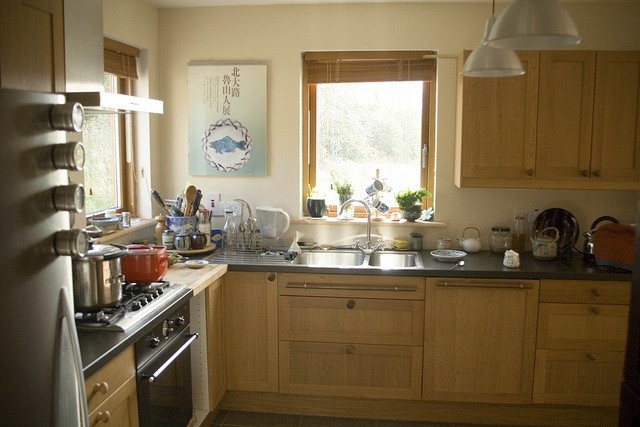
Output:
[291,249,367,271]
[367,249,425,271]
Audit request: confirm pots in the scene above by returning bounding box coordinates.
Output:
[72,240,128,312]
[121,243,169,283]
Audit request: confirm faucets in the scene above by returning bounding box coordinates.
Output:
[338,199,372,249]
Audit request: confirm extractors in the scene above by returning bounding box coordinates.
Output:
[63,0,164,114]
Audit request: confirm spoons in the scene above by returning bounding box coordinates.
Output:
[182,184,197,216]
[248,228,261,250]
[448,259,465,270]
[238,222,246,250]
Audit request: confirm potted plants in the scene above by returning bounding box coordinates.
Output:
[333,179,354,217]
[306,182,325,217]
[395,185,432,222]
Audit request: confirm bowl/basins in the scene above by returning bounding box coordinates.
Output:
[89,216,122,235]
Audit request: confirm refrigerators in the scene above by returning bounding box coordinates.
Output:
[0,87,90,426]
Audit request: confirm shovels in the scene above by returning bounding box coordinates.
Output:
[184,185,196,216]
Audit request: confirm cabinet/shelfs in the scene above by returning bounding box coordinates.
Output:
[534,48,640,189]
[425,277,540,424]
[83,344,139,425]
[532,279,632,426]
[454,49,539,190]
[222,272,278,414]
[277,272,424,423]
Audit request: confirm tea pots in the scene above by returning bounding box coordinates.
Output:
[583,215,621,262]
[456,226,482,252]
[529,226,560,260]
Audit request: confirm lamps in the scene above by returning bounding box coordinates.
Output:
[485,0,582,49]
[457,0,526,78]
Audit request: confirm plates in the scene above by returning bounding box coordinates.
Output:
[529,206,580,253]
[185,259,209,268]
[430,249,467,262]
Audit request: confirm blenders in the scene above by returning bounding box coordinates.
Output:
[252,206,292,250]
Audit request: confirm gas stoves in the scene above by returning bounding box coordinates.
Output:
[73,277,187,331]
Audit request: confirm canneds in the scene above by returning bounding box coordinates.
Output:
[162,230,175,249]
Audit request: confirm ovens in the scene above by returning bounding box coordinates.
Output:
[133,299,193,426]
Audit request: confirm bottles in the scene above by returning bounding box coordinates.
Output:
[122,211,130,228]
[488,225,513,254]
[116,213,123,229]
[511,215,526,255]
[221,210,237,255]
[195,208,211,246]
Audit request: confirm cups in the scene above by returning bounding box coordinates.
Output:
[436,237,447,249]
[410,231,423,250]
[365,177,384,196]
[372,198,390,213]
